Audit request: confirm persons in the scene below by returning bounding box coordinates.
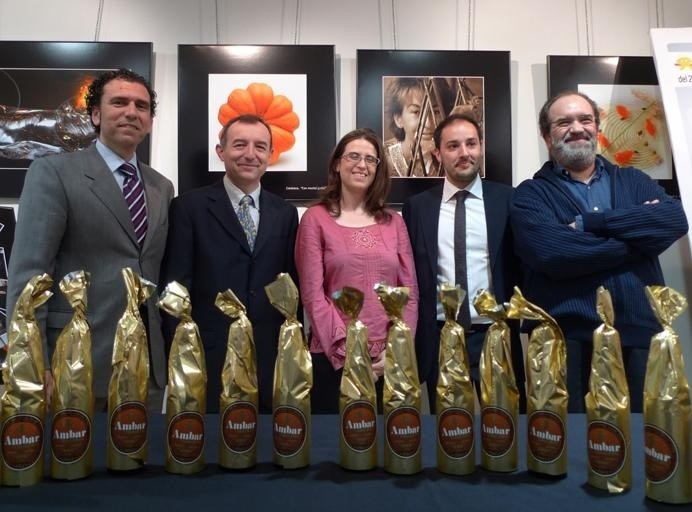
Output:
[295,129,418,414]
[384,77,451,178]
[401,112,527,414]
[157,115,300,413]
[509,90,688,413]
[6,69,174,414]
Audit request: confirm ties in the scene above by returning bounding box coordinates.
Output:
[117,163,148,252]
[236,194,258,255]
[453,189,471,332]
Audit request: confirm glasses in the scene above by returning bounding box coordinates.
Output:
[338,152,382,167]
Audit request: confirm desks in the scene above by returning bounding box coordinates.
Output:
[0,412,692,512]
